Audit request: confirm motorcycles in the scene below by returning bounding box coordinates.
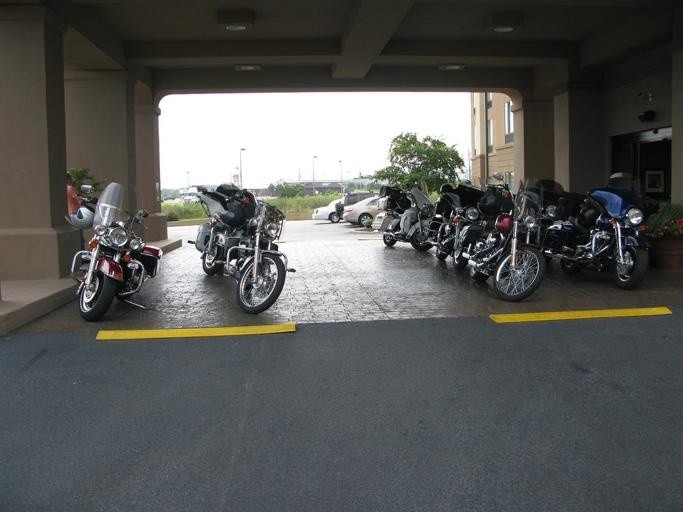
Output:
[187,183,269,271]
[376,186,440,252]
[71,182,163,322]
[503,179,587,266]
[462,172,574,302]
[194,188,296,315]
[541,172,659,290]
[422,182,503,269]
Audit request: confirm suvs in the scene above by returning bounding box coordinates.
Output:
[335,191,380,220]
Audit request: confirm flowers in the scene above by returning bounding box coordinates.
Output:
[643,201,683,240]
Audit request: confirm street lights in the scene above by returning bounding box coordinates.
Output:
[338,160,342,195]
[312,156,318,196]
[239,148,246,190]
[235,166,242,190]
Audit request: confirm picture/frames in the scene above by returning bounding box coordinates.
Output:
[644,169,666,196]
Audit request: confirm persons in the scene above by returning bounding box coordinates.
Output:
[65,170,82,215]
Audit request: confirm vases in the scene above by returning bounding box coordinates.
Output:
[650,236,682,270]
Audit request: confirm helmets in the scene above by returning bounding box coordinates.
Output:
[396,195,411,210]
[214,203,245,225]
[477,191,498,215]
[64,206,95,229]
[440,184,452,192]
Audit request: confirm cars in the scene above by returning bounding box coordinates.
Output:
[343,196,383,227]
[370,204,415,231]
[312,198,345,223]
